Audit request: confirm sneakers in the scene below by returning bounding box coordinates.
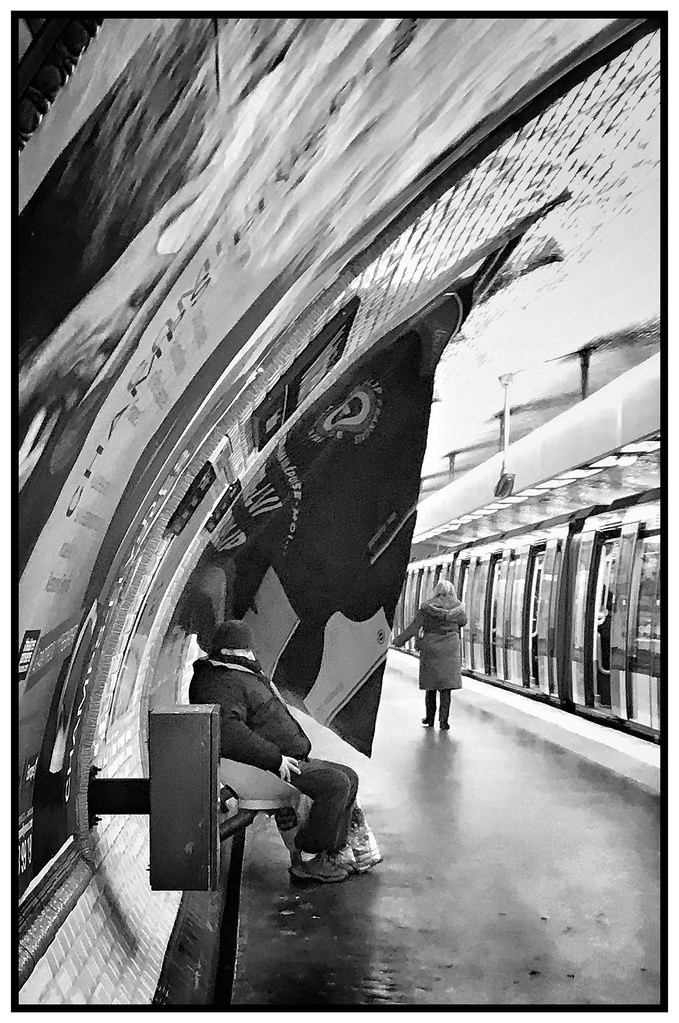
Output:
[288,845,355,883]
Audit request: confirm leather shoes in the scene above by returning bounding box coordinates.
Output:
[421,717,451,730]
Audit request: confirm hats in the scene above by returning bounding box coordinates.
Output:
[214,620,259,650]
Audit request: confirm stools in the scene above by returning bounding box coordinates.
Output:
[217,758,302,868]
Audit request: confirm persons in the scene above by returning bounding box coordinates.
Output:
[390,578,467,730]
[189,618,359,883]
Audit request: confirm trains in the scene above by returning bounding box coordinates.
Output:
[388,488,661,751]
